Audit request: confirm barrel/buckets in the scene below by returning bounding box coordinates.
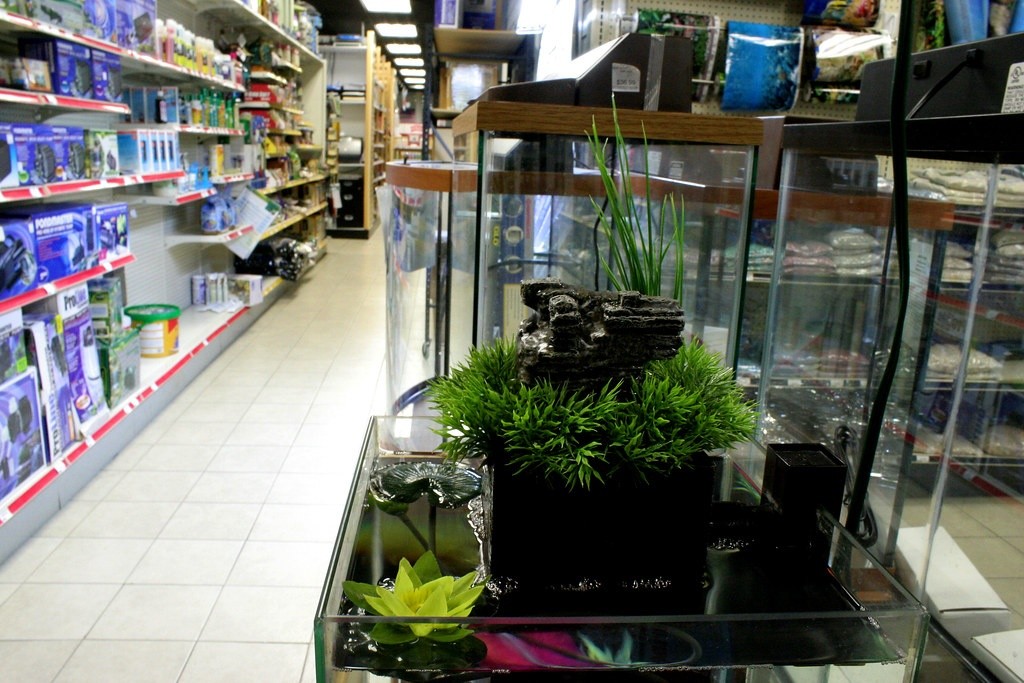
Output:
[125,303,181,358]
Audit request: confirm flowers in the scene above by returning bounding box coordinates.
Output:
[340,549,495,646]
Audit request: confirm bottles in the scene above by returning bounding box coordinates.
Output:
[155,18,249,130]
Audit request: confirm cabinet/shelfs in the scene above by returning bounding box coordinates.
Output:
[607,192,1024,483]
[320,39,376,243]
[0,0,326,563]
[421,26,536,359]
[375,48,394,189]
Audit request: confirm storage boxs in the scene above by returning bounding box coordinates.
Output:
[0,0,267,498]
[315,101,1024,683]
[434,0,508,30]
